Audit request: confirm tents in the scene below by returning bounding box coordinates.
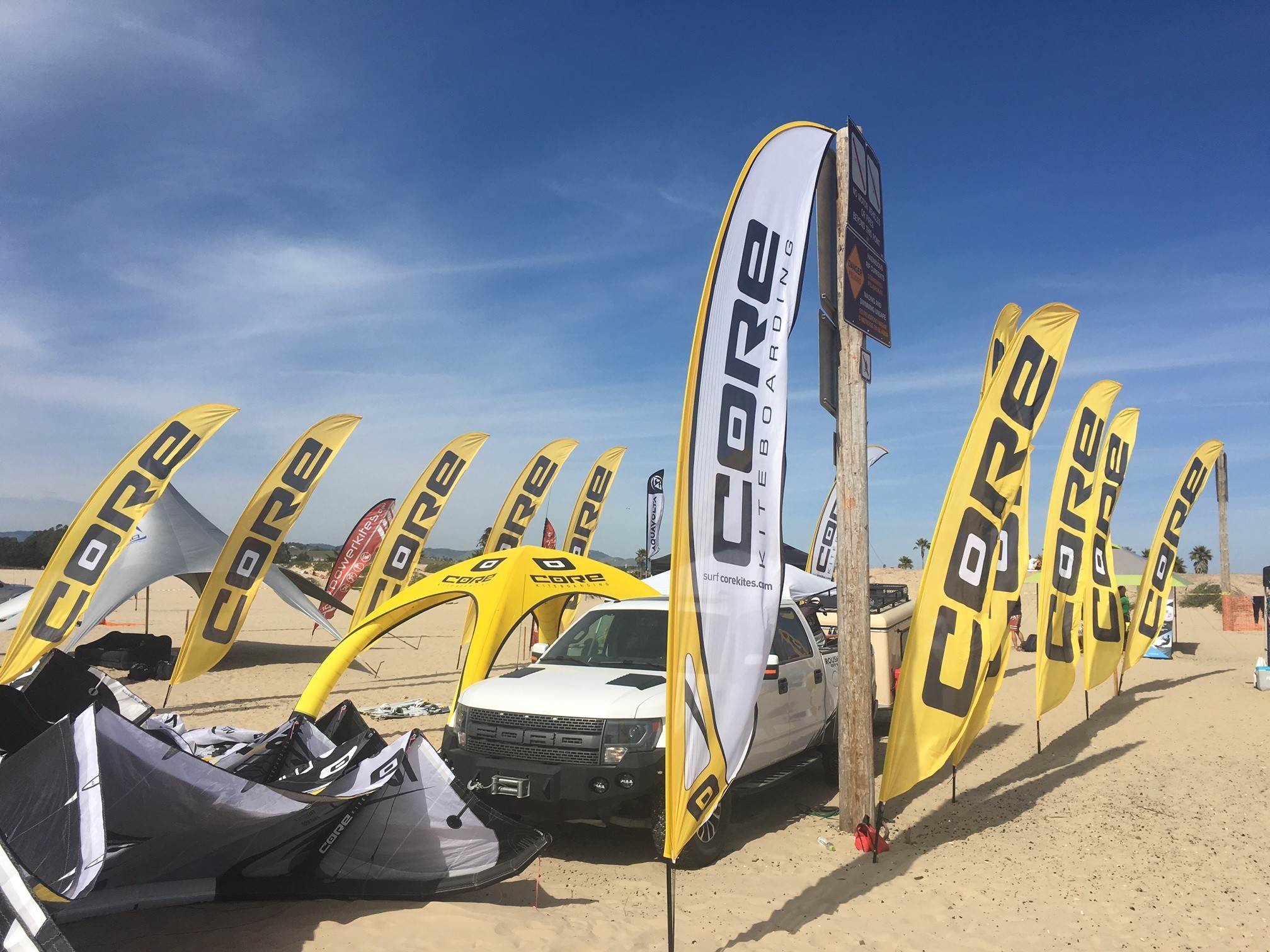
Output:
[1024,572,1194,654]
[633,548,838,653]
[289,546,669,732]
[0,479,385,679]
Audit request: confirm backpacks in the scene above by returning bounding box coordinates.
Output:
[854,814,889,853]
[1023,634,1037,652]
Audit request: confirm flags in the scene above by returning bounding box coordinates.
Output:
[0,119,1223,860]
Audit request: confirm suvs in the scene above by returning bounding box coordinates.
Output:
[435,558,840,830]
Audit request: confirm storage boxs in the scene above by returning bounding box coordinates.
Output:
[1255,666,1270,692]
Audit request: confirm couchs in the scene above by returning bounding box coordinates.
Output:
[75,631,172,671]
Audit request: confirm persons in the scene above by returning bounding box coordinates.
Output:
[1114,586,1132,652]
[1027,554,1041,571]
[1008,595,1025,650]
